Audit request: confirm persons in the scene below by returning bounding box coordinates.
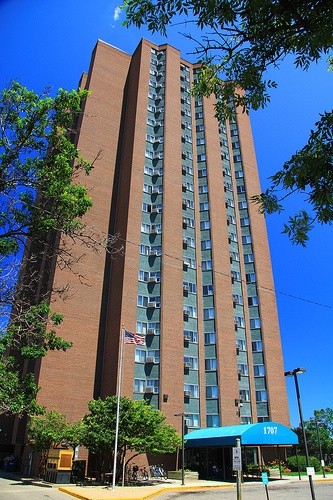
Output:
[0,455,5,470]
[140,466,149,479]
[151,464,164,475]
[133,465,139,480]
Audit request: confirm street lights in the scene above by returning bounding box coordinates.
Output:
[174,412,191,485]
[283,367,316,500]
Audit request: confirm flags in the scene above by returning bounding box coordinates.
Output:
[124,329,146,346]
